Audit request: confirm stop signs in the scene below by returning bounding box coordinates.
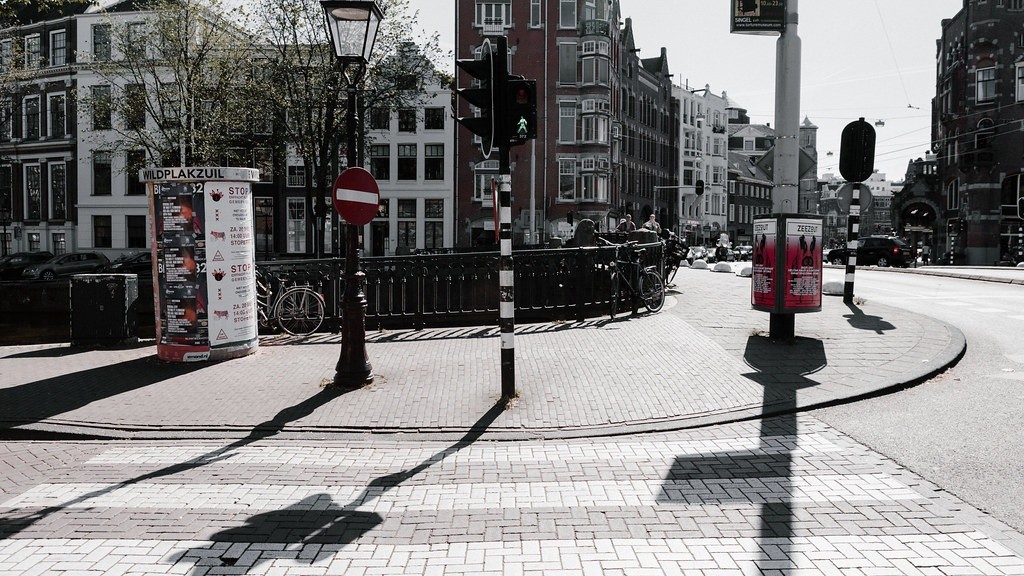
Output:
[333,167,381,228]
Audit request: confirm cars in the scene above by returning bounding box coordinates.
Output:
[827,234,912,267]
[0,249,55,281]
[685,245,753,263]
[94,251,152,281]
[22,251,110,282]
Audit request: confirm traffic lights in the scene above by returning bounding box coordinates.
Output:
[508,78,538,140]
[450,37,496,161]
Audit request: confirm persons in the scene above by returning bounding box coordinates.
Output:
[616,213,662,234]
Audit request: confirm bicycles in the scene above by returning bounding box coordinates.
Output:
[663,227,690,285]
[597,236,666,322]
[254,264,326,337]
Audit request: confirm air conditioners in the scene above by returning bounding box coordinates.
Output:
[598,102,610,112]
[613,126,623,140]
[598,161,610,170]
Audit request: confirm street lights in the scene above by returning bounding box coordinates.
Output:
[317,0,387,389]
